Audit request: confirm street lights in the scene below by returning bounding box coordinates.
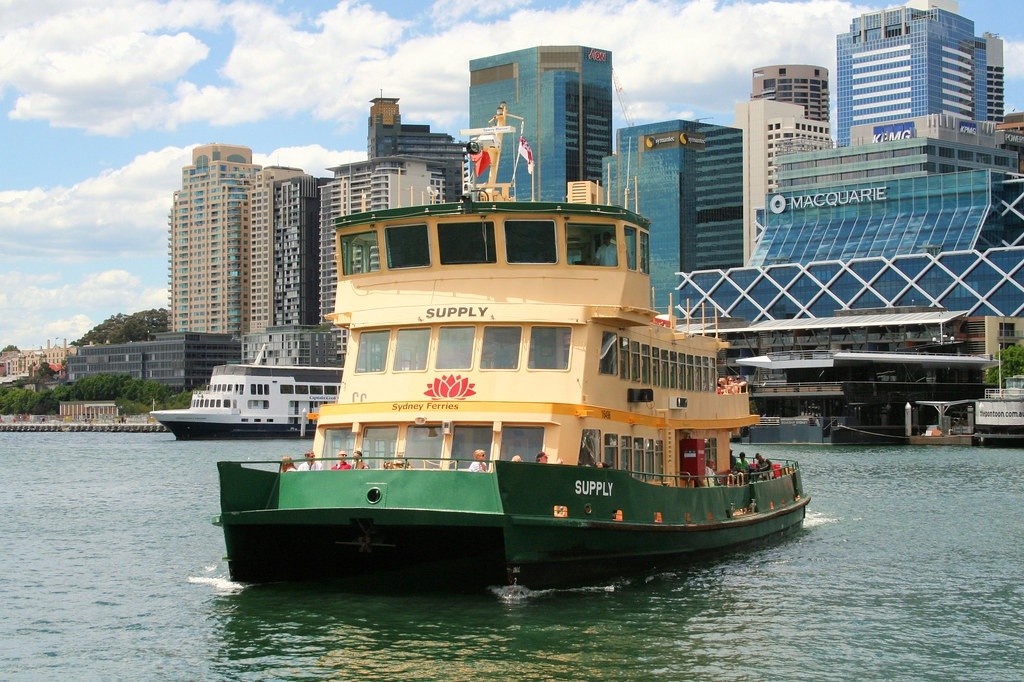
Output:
[905,402,912,437]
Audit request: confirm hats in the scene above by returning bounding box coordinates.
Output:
[337,450,348,457]
[535,452,549,458]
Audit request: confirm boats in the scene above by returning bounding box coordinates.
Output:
[150,344,380,441]
[212,101,811,591]
[973,374,1024,443]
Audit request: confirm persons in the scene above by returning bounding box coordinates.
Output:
[594,462,610,468]
[592,231,618,266]
[352,452,369,469]
[705,459,722,484]
[735,452,750,472]
[468,450,488,472]
[512,456,521,462]
[536,452,548,463]
[298,451,324,470]
[718,375,746,393]
[281,456,298,471]
[331,452,351,470]
[755,454,772,480]
[729,448,736,469]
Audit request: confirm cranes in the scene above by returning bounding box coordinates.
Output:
[611,66,637,127]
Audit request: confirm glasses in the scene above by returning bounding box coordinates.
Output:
[480,454,484,457]
[736,378,741,380]
[304,453,310,458]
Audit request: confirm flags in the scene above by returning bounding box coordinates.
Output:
[518,138,534,173]
